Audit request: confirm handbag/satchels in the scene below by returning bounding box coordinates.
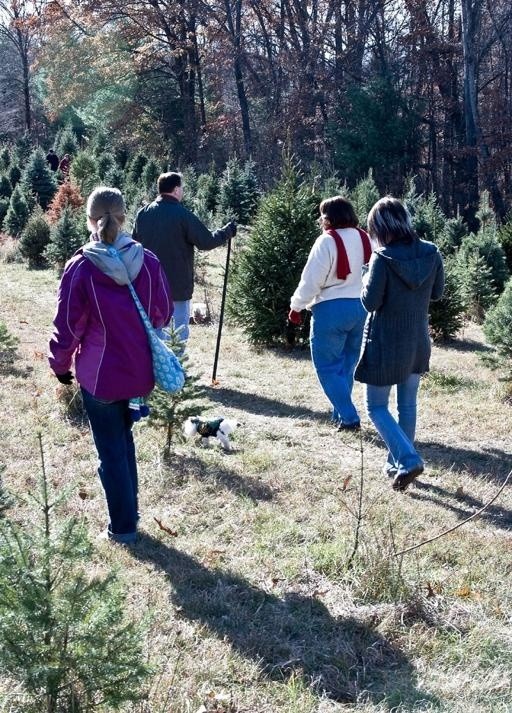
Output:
[149,336,190,398]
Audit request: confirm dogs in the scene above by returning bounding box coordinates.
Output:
[183,415,237,451]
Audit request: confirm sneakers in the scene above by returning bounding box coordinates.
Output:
[386,461,425,492]
[336,420,362,435]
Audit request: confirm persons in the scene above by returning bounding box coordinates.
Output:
[47,148,60,170]
[130,170,238,361]
[58,152,72,173]
[286,194,378,432]
[46,185,176,540]
[350,194,446,494]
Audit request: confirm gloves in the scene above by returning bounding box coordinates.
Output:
[226,223,238,237]
[55,370,75,386]
[289,308,304,326]
